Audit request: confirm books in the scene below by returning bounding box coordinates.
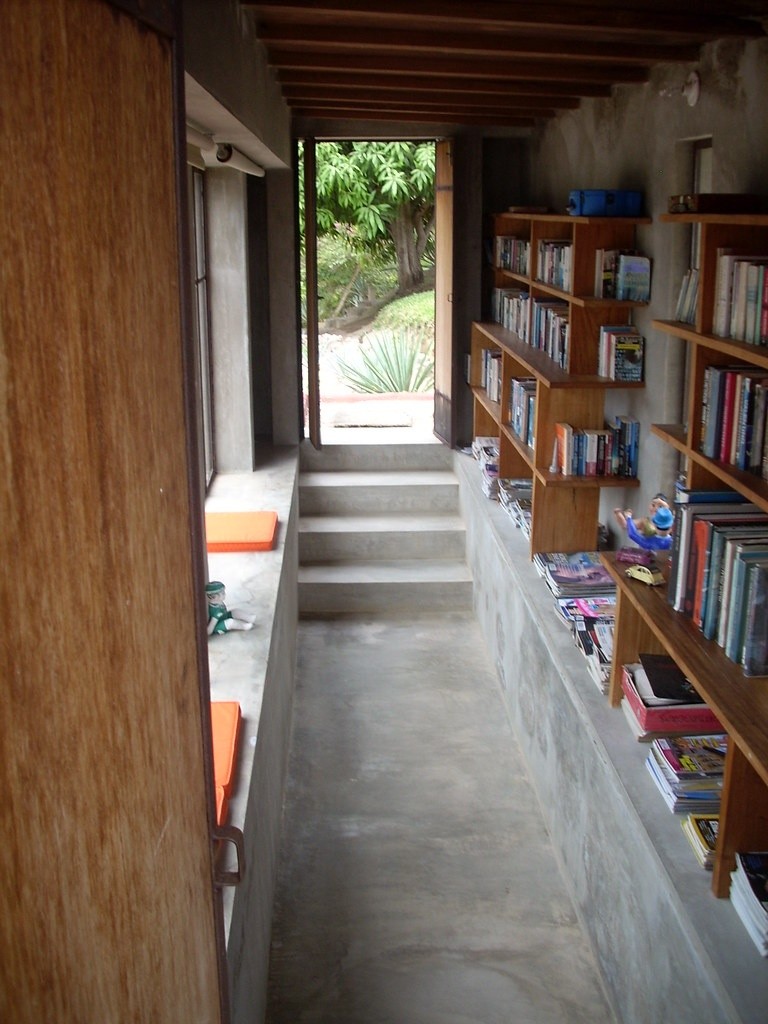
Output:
[467,232,768,957]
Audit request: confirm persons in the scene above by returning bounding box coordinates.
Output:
[613,493,670,551]
[205,581,256,636]
[624,505,674,579]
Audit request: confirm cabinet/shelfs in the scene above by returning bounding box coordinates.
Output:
[467,204,654,564]
[598,213,767,900]
